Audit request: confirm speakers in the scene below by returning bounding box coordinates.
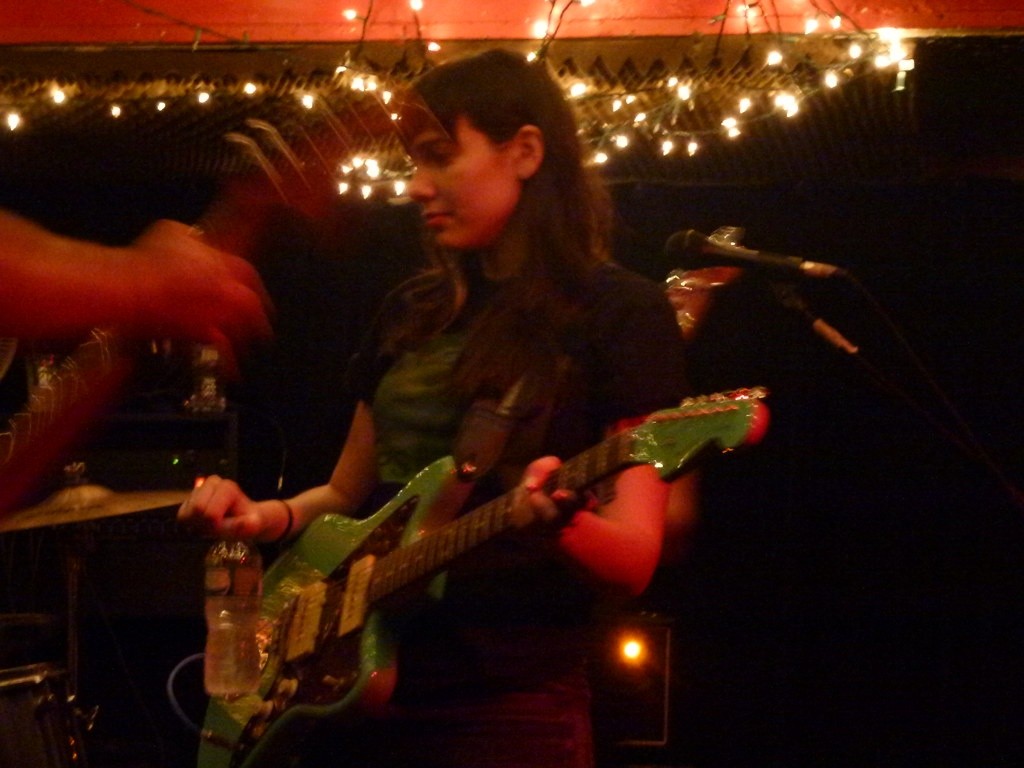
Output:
[0,660,89,768]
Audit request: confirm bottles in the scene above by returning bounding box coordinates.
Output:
[199,531,260,703]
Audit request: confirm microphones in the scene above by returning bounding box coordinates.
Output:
[665,233,850,284]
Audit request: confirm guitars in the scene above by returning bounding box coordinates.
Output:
[4,110,376,498]
[191,379,772,766]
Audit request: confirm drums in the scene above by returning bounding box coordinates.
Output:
[0,659,101,768]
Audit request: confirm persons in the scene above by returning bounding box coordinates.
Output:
[177,47,689,768]
[1,217,275,524]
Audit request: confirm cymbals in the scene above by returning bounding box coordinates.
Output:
[0,485,194,537]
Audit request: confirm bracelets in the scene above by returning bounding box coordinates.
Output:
[273,498,294,544]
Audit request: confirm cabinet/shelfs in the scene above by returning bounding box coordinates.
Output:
[0,411,248,623]
